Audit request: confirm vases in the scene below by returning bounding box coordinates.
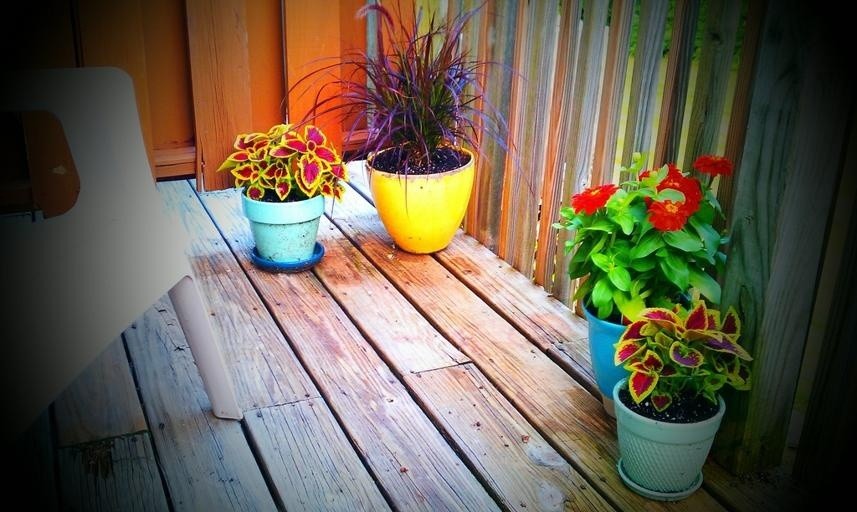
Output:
[551,149,733,318]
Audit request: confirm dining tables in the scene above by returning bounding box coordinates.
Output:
[280,0,538,256]
[612,299,754,503]
[216,122,348,271]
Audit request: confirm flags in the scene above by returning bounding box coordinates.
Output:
[580,301,629,419]
